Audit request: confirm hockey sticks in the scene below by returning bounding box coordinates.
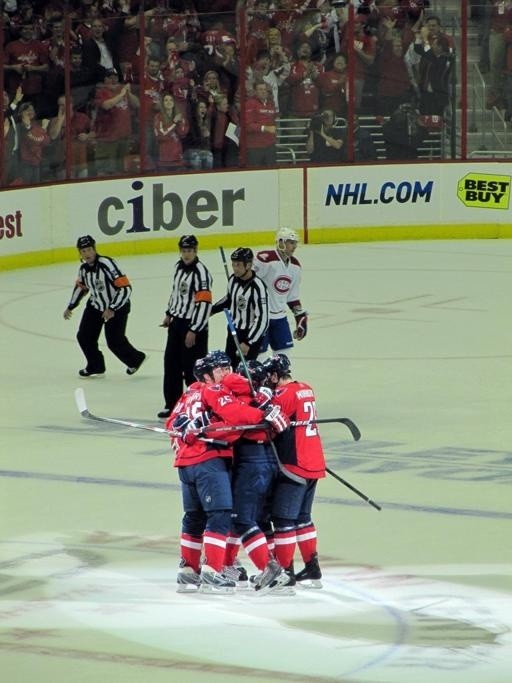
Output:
[204,417,361,442]
[74,387,230,447]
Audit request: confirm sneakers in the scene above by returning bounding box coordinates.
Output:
[177,558,202,583]
[127,351,146,375]
[157,408,172,417]
[233,559,248,580]
[295,552,321,580]
[271,570,290,589]
[200,564,236,588]
[284,559,296,586]
[79,369,105,377]
[255,561,281,590]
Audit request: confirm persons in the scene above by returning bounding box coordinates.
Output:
[471,1,512,120]
[339,115,377,163]
[76,5,98,47]
[185,98,215,170]
[209,248,269,373]
[157,235,213,418]
[16,101,50,184]
[244,0,348,67]
[206,92,235,168]
[91,68,142,177]
[305,110,347,164]
[285,41,323,165]
[66,236,147,376]
[245,47,291,143]
[1,87,32,189]
[251,227,307,364]
[49,96,95,180]
[0,0,81,98]
[78,0,130,87]
[356,1,453,117]
[153,92,190,175]
[320,54,349,117]
[383,104,430,162]
[167,350,325,596]
[119,0,238,99]
[240,80,278,167]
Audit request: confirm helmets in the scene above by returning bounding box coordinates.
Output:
[194,357,218,382]
[76,235,95,250]
[237,360,264,382]
[276,228,299,242]
[263,354,290,380]
[178,235,198,248]
[209,352,232,367]
[230,247,253,262]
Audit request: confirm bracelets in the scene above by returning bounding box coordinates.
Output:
[172,120,177,124]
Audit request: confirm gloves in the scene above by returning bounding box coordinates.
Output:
[263,408,290,432]
[173,416,187,433]
[182,411,210,445]
[418,114,442,128]
[295,309,308,341]
[251,386,273,406]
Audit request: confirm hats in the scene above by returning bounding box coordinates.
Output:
[105,67,118,76]
